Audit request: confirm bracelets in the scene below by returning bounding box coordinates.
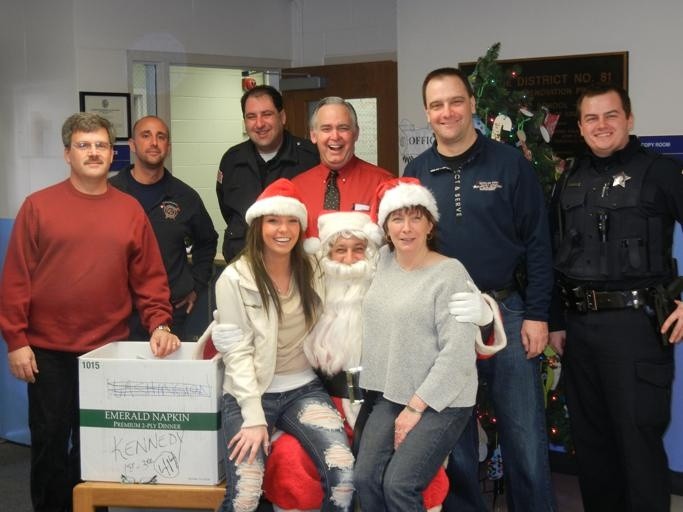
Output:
[405,401,423,418]
[152,323,172,331]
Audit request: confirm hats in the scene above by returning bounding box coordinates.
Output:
[244,176,321,256]
[369,177,440,248]
[311,206,384,251]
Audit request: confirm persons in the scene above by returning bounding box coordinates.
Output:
[390,67,564,511]
[347,178,480,510]
[280,94,395,222]
[105,116,220,346]
[191,207,509,512]
[215,83,319,270]
[536,77,681,511]
[208,177,356,511]
[1,111,181,511]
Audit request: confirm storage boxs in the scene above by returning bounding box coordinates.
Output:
[74,337,231,485]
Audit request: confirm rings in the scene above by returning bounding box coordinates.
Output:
[262,441,270,447]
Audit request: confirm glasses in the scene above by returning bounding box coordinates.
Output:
[70,140,113,152]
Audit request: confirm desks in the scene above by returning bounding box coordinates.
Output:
[72,478,230,512]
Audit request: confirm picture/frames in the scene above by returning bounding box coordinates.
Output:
[77,90,132,142]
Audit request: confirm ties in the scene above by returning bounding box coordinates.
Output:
[323,171,341,213]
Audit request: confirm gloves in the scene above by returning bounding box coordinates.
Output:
[210,309,243,355]
[448,279,493,328]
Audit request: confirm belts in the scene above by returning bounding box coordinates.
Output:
[560,282,654,313]
[311,365,369,404]
[486,286,516,303]
